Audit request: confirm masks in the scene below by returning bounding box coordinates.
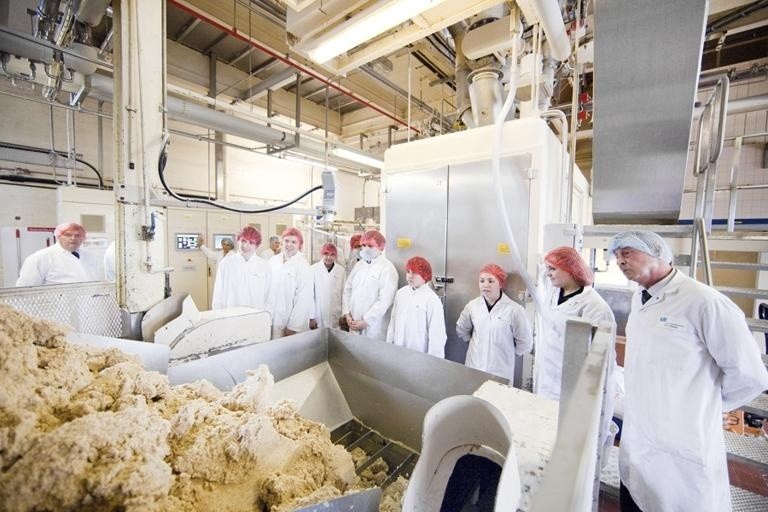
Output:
[352,246,380,261]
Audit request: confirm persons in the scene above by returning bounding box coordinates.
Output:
[344,234,362,279]
[341,230,398,342]
[533,246,616,469]
[309,243,346,332]
[211,224,269,312]
[385,257,447,359]
[258,236,281,260]
[610,229,767,512]
[455,263,532,388]
[196,234,235,268]
[267,227,312,340]
[15,221,97,289]
